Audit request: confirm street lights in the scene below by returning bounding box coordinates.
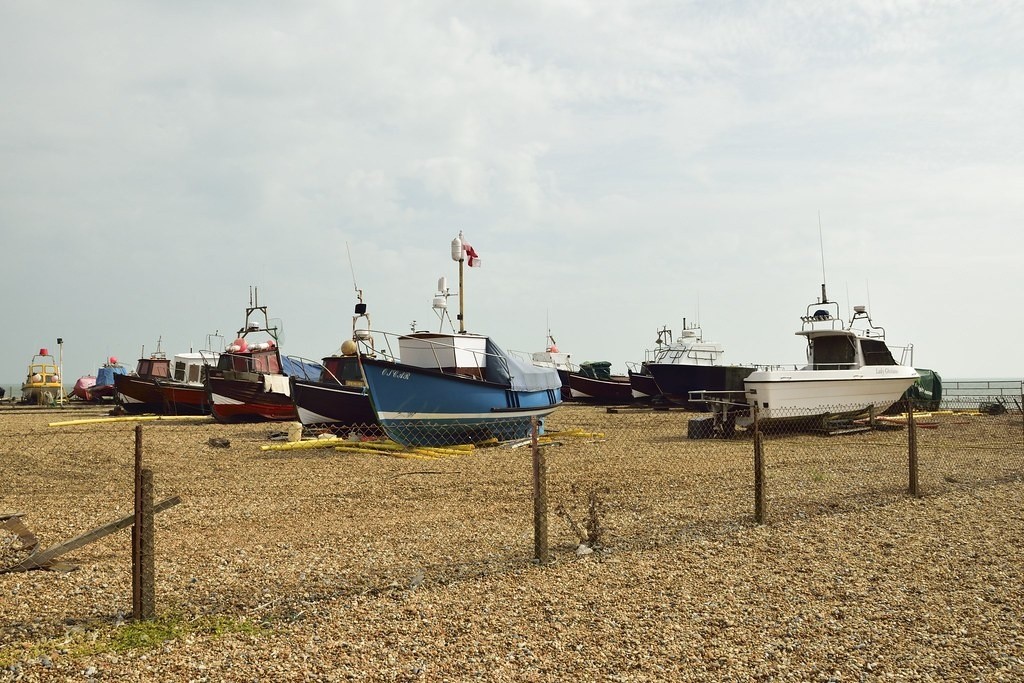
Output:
[57,338,63,409]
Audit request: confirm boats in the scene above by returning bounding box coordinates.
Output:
[20,349,63,405]
[74,210,942,447]
